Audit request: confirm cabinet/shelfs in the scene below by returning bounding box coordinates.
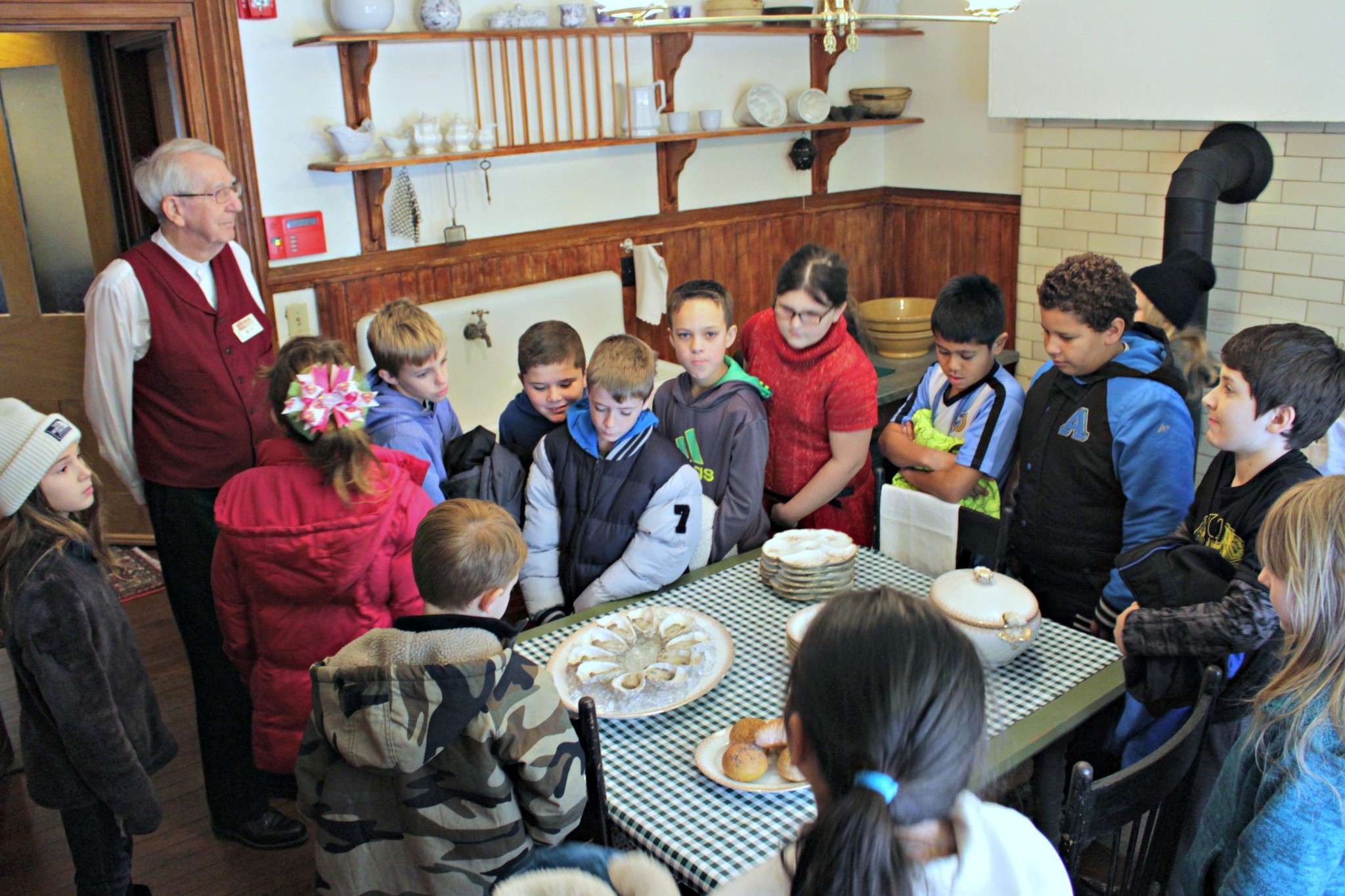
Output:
[294,24,925,249]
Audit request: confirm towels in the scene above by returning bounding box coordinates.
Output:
[633,245,670,325]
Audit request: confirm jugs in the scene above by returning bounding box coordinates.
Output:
[617,79,667,138]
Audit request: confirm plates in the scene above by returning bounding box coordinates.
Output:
[786,602,827,665]
[760,528,859,600]
[695,726,811,792]
[545,607,734,718]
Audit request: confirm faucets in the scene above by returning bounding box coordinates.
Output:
[464,309,495,347]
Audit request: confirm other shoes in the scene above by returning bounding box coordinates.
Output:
[126,883,152,896]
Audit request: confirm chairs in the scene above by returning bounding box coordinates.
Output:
[1059,665,1220,896]
[875,465,1013,581]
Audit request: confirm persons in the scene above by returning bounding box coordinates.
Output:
[738,244,879,551]
[971,252,1203,882]
[650,278,770,570]
[876,272,1025,573]
[79,138,311,851]
[1163,477,1345,896]
[0,397,179,895]
[359,297,465,513]
[517,335,703,629]
[705,583,1078,896]
[1112,324,1345,883]
[296,496,587,896]
[211,333,425,800]
[1127,250,1220,475]
[498,320,588,482]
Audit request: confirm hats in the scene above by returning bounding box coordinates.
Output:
[0,397,81,519]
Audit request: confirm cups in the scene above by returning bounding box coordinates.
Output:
[698,109,723,131]
[669,6,691,19]
[476,124,496,150]
[381,129,412,159]
[559,3,590,29]
[786,88,831,124]
[667,111,690,133]
[326,118,376,164]
[733,84,788,128]
[592,4,618,28]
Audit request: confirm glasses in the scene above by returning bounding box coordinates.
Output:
[172,182,242,204]
[773,296,835,328]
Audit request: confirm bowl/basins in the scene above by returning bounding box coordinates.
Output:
[856,297,937,358]
[330,0,395,34]
[927,565,1042,668]
[764,6,814,28]
[849,87,912,119]
[420,0,461,32]
[702,0,765,27]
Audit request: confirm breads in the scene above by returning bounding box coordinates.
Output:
[777,747,807,781]
[754,717,788,749]
[730,718,770,754]
[722,742,769,781]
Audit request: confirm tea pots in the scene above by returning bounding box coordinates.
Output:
[411,113,442,154]
[445,115,476,153]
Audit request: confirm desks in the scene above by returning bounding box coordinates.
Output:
[510,532,1123,894]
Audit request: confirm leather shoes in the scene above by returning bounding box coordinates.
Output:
[212,801,308,847]
[259,774,323,798]
